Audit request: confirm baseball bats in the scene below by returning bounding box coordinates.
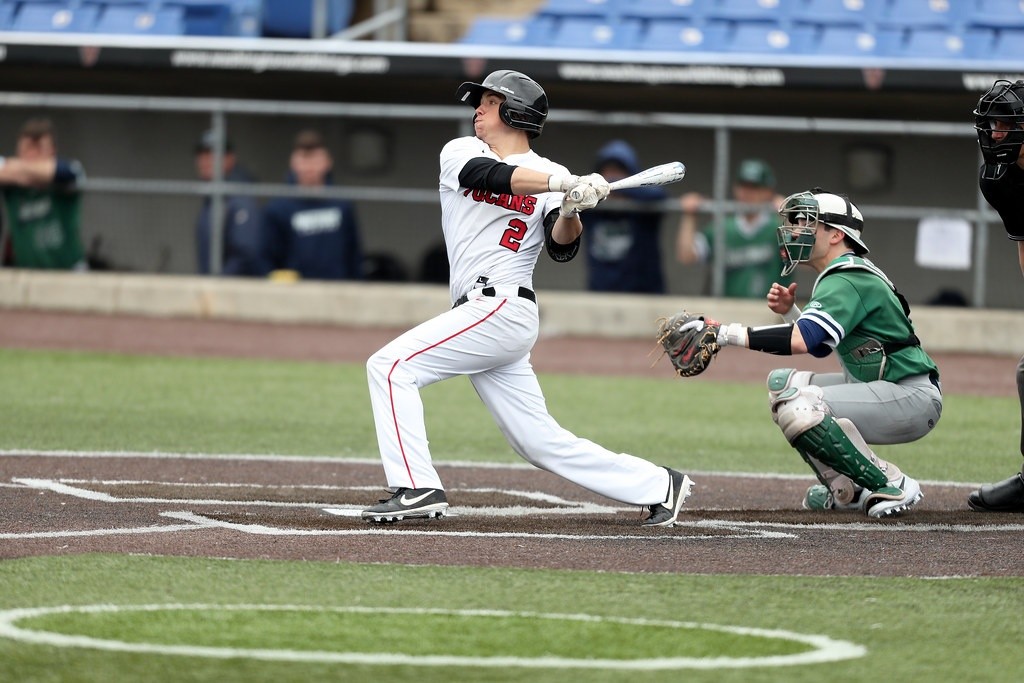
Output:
[569,161,686,202]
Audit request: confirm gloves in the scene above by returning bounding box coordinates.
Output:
[559,184,599,217]
[547,172,611,202]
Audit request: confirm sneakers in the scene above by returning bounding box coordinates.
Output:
[806,484,863,512]
[640,467,695,528]
[867,474,924,518]
[361,486,450,522]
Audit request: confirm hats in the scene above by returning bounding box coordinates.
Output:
[596,139,637,176]
[195,127,232,156]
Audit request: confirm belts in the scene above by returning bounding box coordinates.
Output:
[452,287,537,305]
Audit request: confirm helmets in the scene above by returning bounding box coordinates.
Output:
[794,190,871,254]
[455,71,547,139]
[974,79,1023,181]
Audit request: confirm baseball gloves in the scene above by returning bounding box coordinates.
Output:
[657,312,723,377]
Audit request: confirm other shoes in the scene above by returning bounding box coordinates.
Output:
[967,473,1023,512]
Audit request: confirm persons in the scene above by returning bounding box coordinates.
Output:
[0,118,89,275]
[677,158,794,299]
[193,126,268,277]
[357,69,695,527]
[680,187,942,518]
[964,79,1024,512]
[582,141,670,294]
[268,130,362,283]
